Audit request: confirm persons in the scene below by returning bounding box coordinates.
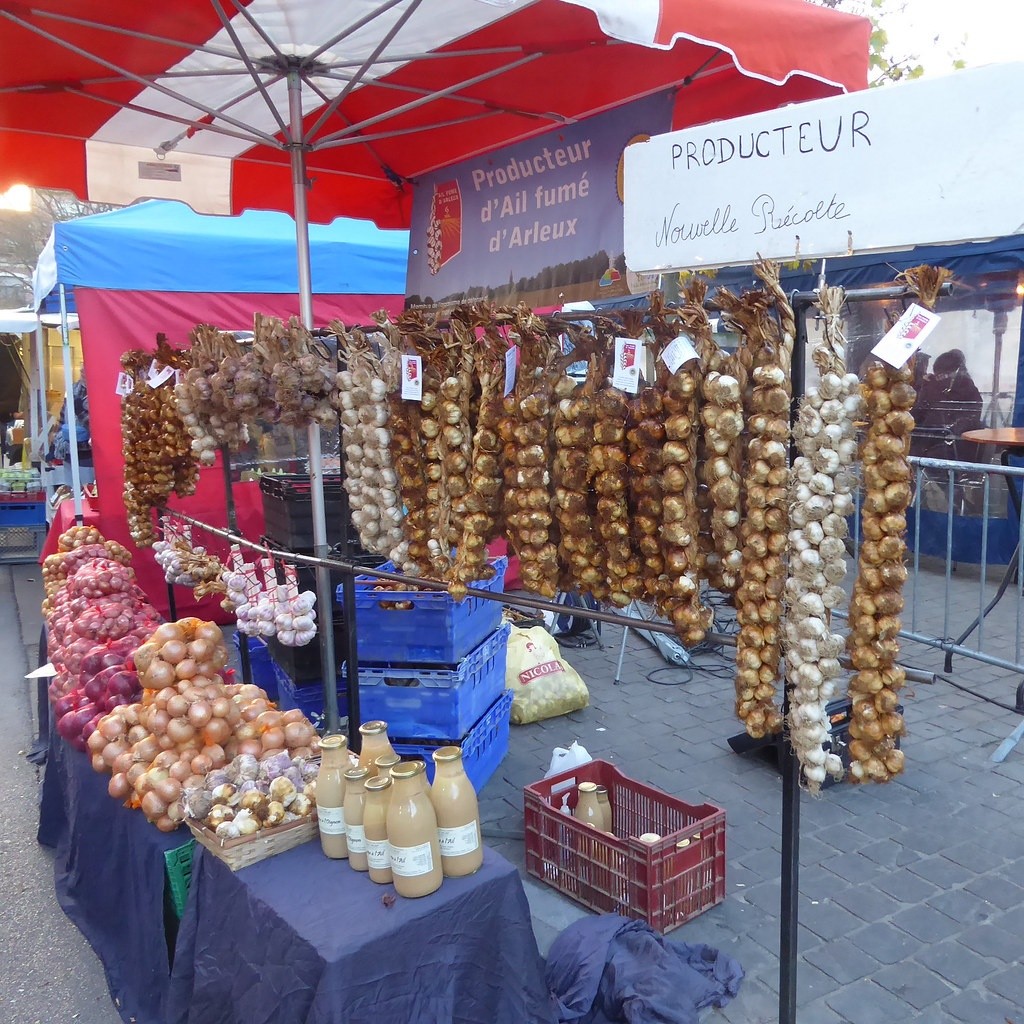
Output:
[909,349,984,515]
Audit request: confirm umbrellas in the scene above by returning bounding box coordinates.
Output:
[0,0,871,737]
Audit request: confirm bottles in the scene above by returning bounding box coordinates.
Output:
[431,746,484,878]
[595,785,612,834]
[575,782,604,859]
[315,720,442,898]
[636,832,702,911]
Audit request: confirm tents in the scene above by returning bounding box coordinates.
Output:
[0,199,564,627]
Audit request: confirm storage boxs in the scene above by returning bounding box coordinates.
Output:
[0,501,46,564]
[523,757,726,935]
[165,837,198,919]
[232,474,510,793]
[83,481,99,510]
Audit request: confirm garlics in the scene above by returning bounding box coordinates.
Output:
[182,754,319,838]
[119,347,916,786]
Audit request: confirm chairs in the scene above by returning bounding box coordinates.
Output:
[910,425,977,514]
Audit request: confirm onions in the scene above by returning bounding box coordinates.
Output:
[40,524,314,832]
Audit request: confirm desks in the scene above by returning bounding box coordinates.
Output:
[961,427,1024,584]
[38,499,99,563]
